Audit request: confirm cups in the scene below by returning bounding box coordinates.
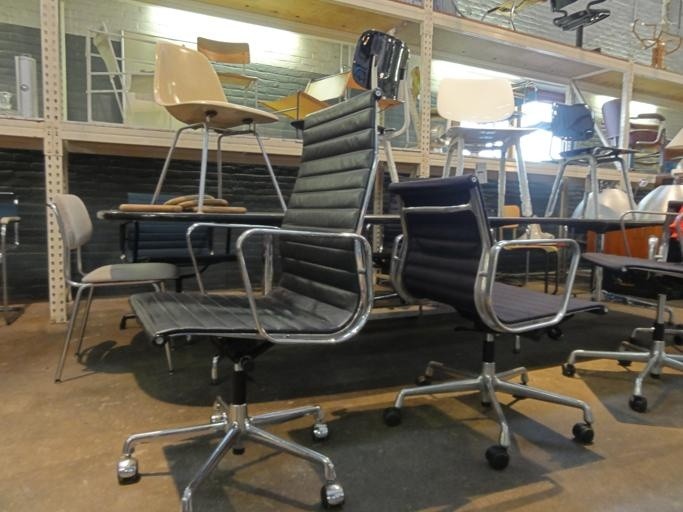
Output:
[0,89,13,113]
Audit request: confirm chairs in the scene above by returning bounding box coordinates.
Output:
[117,85,383,509]
[46,194,180,382]
[98,1,682,384]
[386,174,594,470]
[1,170,32,324]
[563,200,683,412]
